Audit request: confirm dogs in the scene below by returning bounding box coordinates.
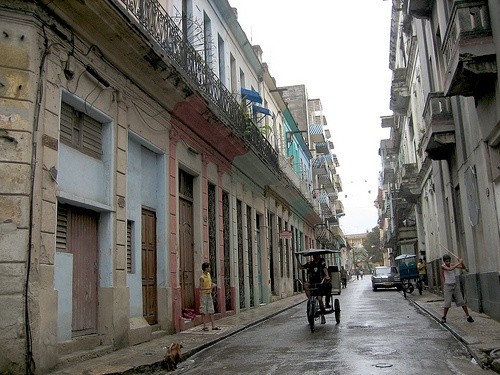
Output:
[165,342,183,372]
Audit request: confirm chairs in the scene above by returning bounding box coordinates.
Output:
[399,262,419,279]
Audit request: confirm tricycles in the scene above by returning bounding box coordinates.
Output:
[394,253,423,300]
[294,248,342,335]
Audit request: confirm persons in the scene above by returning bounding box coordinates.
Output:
[440,254,475,323]
[297,253,332,309]
[199,262,222,331]
[340,266,349,289]
[357,269,364,280]
[287,155,294,166]
[389,252,396,268]
[417,258,427,290]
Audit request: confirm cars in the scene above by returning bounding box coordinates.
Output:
[372,266,402,292]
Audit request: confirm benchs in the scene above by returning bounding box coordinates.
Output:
[307,272,340,293]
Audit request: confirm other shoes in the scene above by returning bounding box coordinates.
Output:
[467,316,474,322]
[321,316,326,324]
[325,304,331,308]
[441,317,446,323]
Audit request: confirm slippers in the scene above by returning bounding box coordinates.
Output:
[203,327,209,331]
[212,326,221,329]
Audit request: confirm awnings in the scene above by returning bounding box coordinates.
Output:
[241,87,274,119]
